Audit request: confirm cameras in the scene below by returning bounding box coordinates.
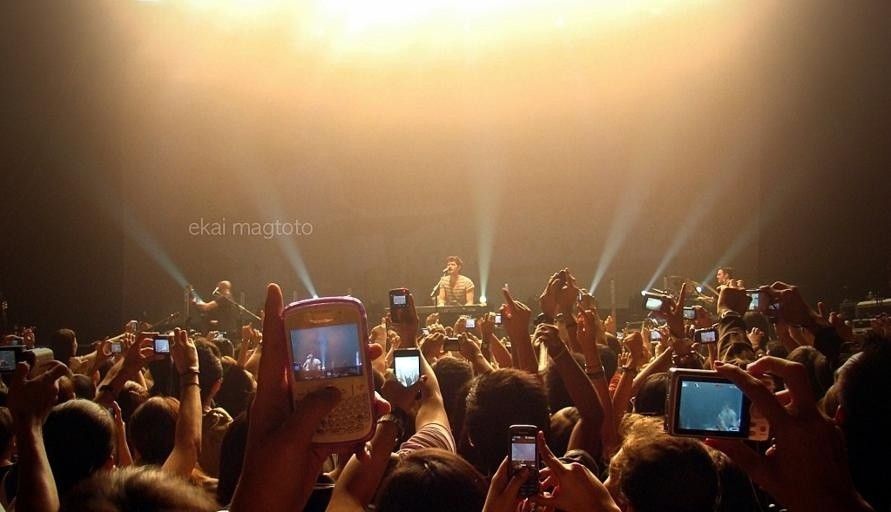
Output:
[664,368,775,442]
[681,307,697,320]
[558,269,567,288]
[389,287,409,324]
[0,331,227,380]
[641,290,671,313]
[507,424,539,499]
[649,329,662,342]
[744,289,781,313]
[693,328,719,344]
[280,296,377,449]
[421,313,503,351]
[392,346,420,388]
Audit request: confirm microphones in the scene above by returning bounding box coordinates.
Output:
[212,286,219,296]
[441,266,451,272]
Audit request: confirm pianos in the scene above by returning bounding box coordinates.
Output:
[384,304,488,317]
